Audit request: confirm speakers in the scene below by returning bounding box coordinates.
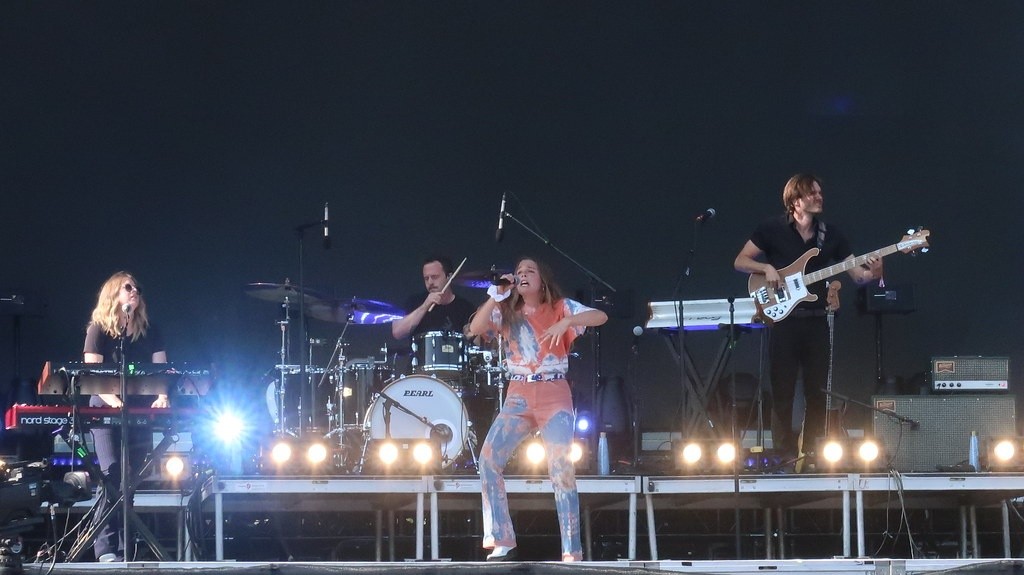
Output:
[870,394,1016,473]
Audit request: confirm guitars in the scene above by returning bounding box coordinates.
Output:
[747,224,930,322]
[794,280,842,474]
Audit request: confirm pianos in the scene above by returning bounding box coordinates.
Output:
[6,362,217,562]
[643,298,774,437]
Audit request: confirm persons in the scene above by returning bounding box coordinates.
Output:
[470,256,608,563]
[393,253,495,471]
[734,172,883,474]
[83,270,172,562]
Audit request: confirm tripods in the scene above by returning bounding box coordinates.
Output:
[318,308,365,468]
[271,295,301,440]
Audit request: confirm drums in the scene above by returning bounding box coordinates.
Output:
[339,365,395,429]
[279,367,336,440]
[410,330,470,373]
[363,374,470,469]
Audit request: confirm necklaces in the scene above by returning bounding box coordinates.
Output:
[521,304,538,315]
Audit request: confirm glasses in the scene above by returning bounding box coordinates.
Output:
[120,284,143,295]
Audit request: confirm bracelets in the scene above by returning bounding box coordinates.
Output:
[487,284,511,302]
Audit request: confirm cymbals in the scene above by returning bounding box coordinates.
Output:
[305,298,403,324]
[239,283,328,305]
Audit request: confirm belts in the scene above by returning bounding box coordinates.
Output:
[510,373,566,382]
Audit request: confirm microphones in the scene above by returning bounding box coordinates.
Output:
[911,421,920,430]
[436,429,449,439]
[496,195,505,243]
[697,208,716,221]
[323,206,330,249]
[492,277,520,285]
[122,303,133,314]
[632,327,643,351]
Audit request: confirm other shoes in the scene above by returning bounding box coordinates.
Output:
[487,546,517,561]
[800,464,816,473]
[564,556,582,562]
[98,553,124,562]
[772,463,785,474]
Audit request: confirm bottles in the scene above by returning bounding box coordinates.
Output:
[597,432,610,475]
[969,431,981,473]
